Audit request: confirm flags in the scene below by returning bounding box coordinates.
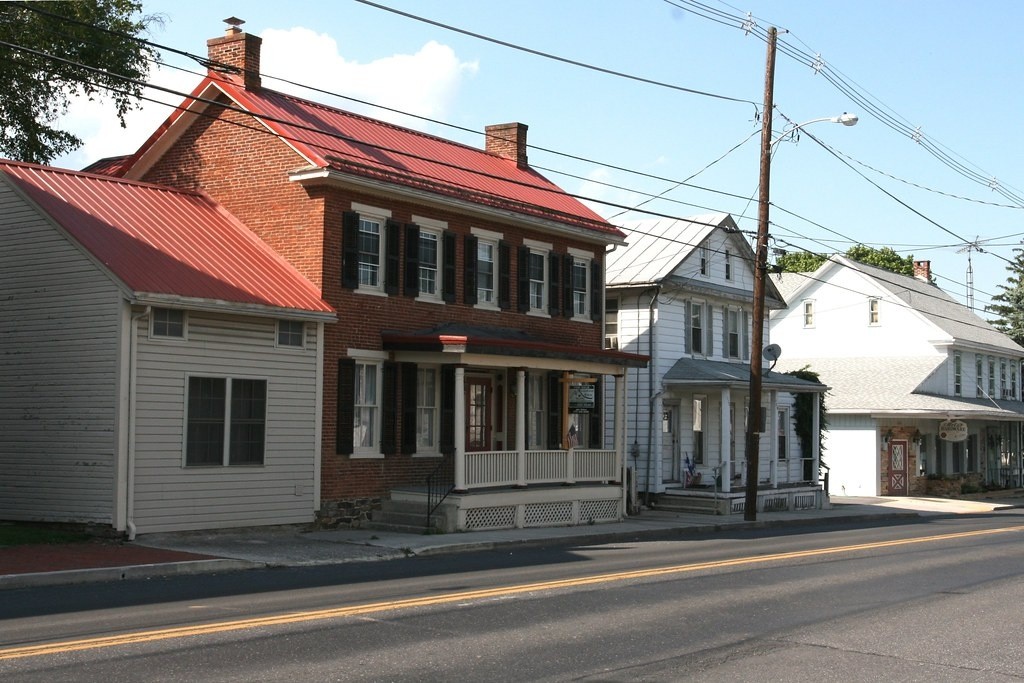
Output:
[566,425,579,448]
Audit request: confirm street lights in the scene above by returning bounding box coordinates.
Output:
[744,113,861,522]
